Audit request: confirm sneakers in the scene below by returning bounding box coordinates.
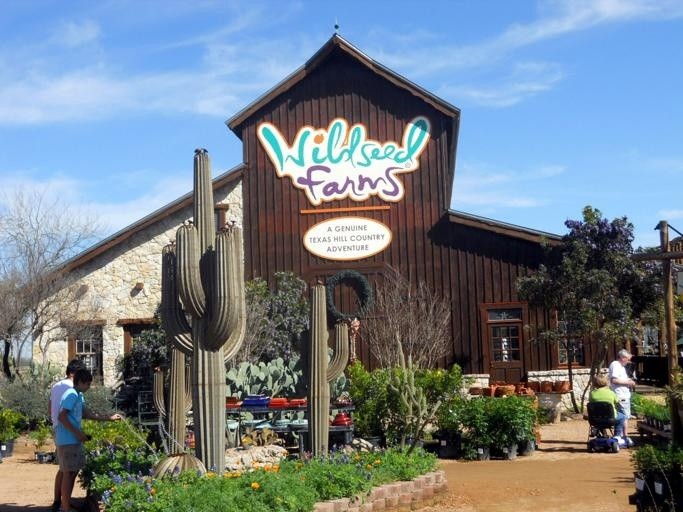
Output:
[613,435,635,447]
[52,500,79,512]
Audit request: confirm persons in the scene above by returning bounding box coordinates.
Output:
[607,348,636,448]
[587,374,628,439]
[52,367,125,511]
[47,358,87,510]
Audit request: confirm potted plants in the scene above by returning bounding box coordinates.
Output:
[1,411,19,463]
[29,418,50,463]
[436,394,539,462]
